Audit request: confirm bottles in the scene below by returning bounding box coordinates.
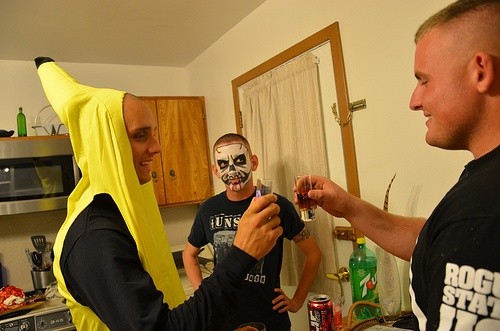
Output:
[349,237,384,320]
[16,107,27,137]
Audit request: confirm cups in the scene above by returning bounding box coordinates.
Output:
[237,322,267,331]
[332,298,344,331]
[256,178,274,219]
[31,271,55,293]
[295,174,319,223]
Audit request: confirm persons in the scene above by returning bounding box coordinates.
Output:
[33,57,283,331]
[182,133,323,331]
[293,0,500,331]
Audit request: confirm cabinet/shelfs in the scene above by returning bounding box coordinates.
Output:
[133,95,215,211]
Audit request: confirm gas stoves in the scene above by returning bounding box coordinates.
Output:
[0,289,76,331]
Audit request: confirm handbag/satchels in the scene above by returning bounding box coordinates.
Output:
[341,301,420,331]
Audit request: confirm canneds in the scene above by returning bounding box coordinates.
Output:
[308,294,334,331]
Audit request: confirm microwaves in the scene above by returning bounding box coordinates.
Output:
[0,139,82,216]
